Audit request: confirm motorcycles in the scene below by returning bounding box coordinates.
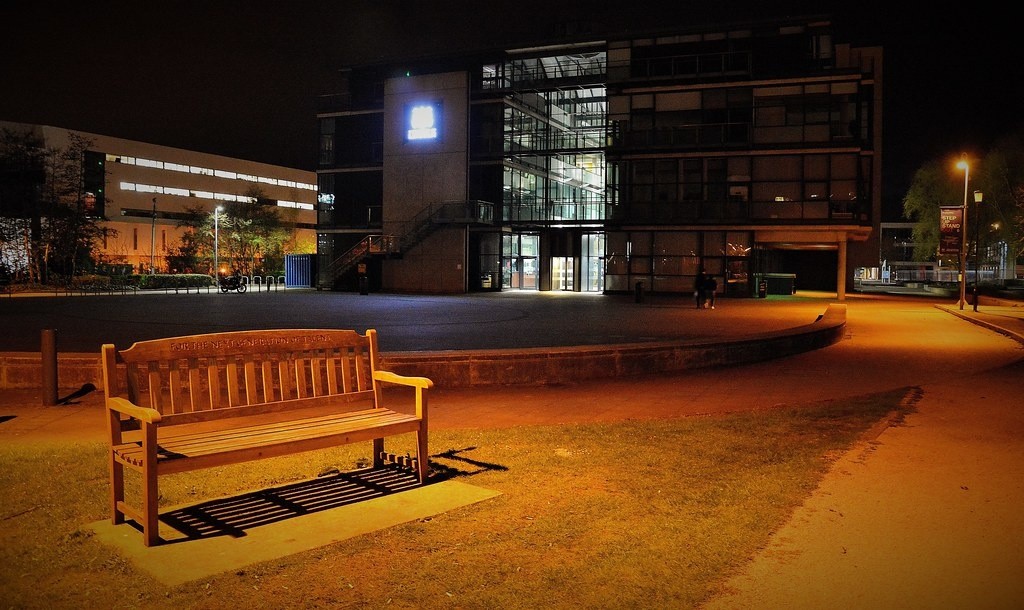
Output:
[220,269,247,294]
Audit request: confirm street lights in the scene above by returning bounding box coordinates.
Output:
[215,206,223,286]
[955,161,969,310]
[973,189,983,312]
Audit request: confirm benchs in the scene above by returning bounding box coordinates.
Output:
[102,328,434,548]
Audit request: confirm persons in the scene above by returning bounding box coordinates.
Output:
[695,266,719,309]
